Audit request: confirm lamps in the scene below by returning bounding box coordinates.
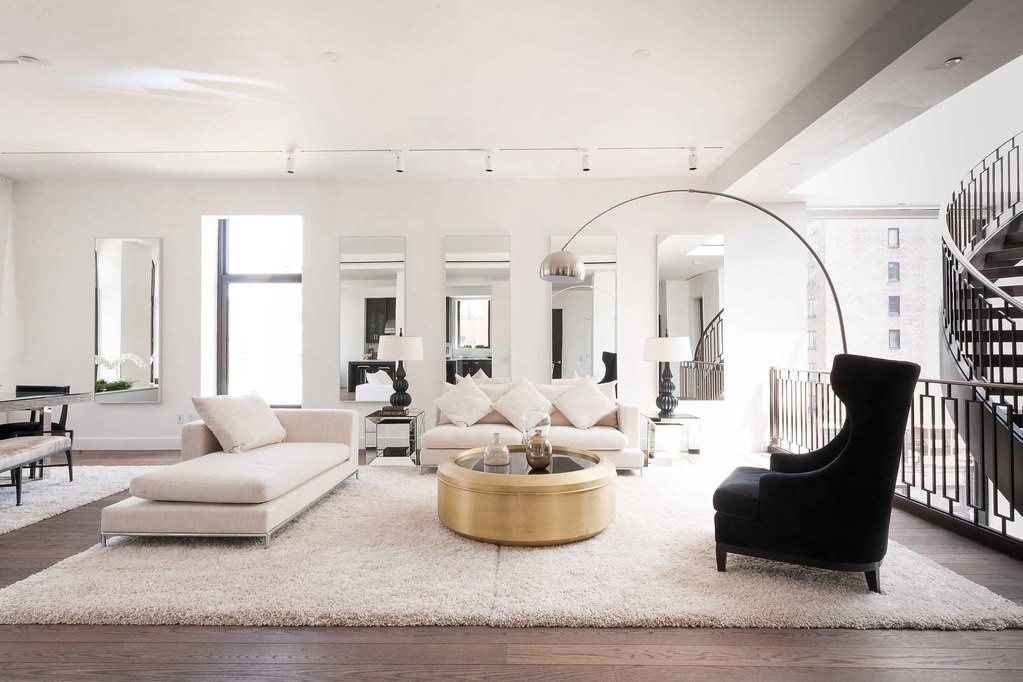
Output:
[643,337,693,418]
[540,189,848,354]
[285,147,698,171]
[377,329,424,414]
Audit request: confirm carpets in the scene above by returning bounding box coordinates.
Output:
[0,457,1023,631]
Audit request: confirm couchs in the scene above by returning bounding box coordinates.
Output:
[100,408,359,550]
[419,379,644,477]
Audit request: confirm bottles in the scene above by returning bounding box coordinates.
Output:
[483,433,510,466]
[525,429,552,470]
[369,347,377,358]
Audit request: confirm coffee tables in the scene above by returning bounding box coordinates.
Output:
[437,442,618,545]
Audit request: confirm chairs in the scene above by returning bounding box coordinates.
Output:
[713,354,921,594]
[9,385,73,483]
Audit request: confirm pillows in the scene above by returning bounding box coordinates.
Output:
[192,392,287,452]
[553,378,619,430]
[491,379,558,433]
[432,374,496,428]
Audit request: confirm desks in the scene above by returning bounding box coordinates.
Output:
[347,360,397,392]
[0,392,92,478]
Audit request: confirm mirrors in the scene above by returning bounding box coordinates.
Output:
[93,237,160,404]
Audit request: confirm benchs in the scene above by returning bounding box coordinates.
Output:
[0,430,74,506]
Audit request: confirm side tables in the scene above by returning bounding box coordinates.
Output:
[645,413,702,460]
[365,408,425,466]
